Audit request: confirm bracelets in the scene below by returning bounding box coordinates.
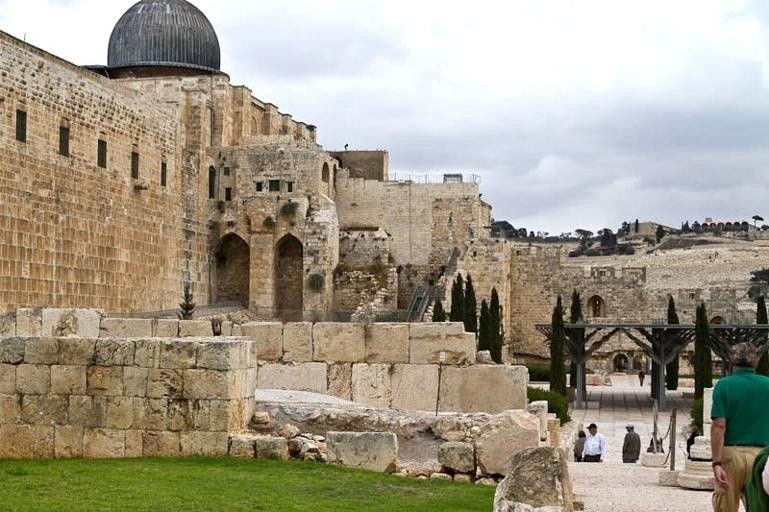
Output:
[712,462,721,467]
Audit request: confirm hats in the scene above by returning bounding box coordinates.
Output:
[585,423,597,429]
[626,423,634,428]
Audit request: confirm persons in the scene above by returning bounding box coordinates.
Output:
[639,371,644,386]
[573,431,586,462]
[622,425,640,463]
[745,446,769,512]
[581,423,605,462]
[711,341,769,512]
